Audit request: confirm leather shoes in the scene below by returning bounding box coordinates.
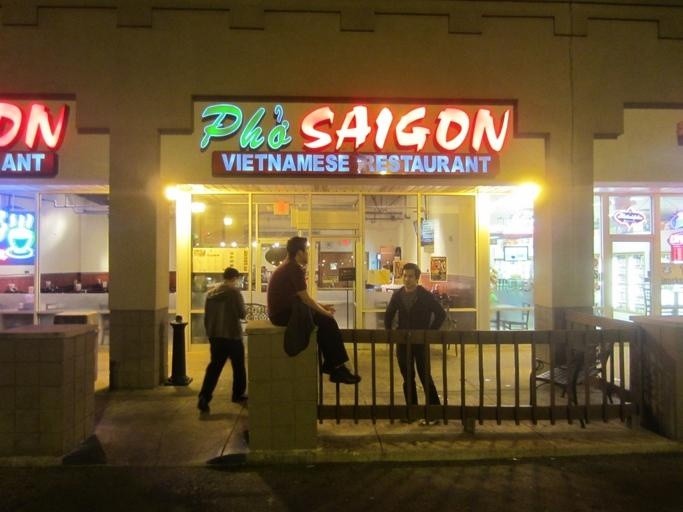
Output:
[196,404,210,412]
[232,395,249,403]
[322,364,361,384]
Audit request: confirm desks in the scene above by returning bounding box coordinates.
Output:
[361,306,477,357]
[492,304,522,332]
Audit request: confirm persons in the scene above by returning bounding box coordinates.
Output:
[267,236,363,384]
[197,267,248,412]
[385,263,446,425]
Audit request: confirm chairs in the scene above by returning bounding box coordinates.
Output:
[98,303,110,346]
[529,342,614,429]
[501,303,530,331]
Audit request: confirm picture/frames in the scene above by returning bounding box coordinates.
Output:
[429,256,447,283]
